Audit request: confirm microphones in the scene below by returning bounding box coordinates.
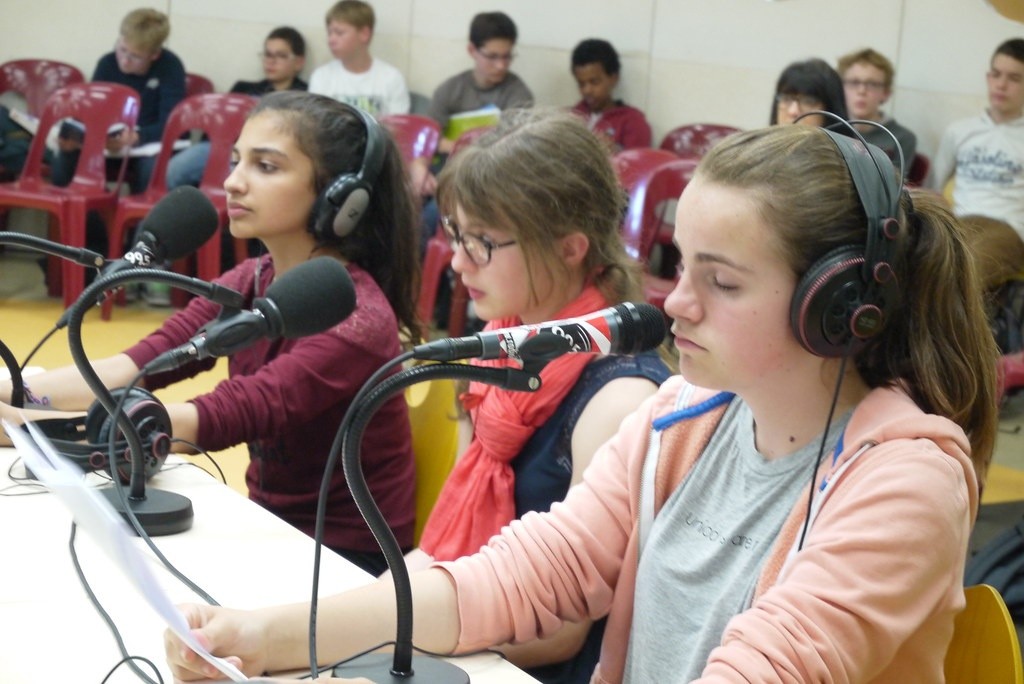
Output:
[144,257,358,374]
[414,301,667,361]
[57,186,219,329]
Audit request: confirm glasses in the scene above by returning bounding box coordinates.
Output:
[478,49,514,62]
[437,218,574,267]
[776,91,822,107]
[258,49,289,60]
[114,39,153,62]
[843,78,885,88]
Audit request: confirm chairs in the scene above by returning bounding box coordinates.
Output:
[944,585,1024,683]
[617,124,745,304]
[99,87,263,320]
[399,361,463,543]
[0,61,84,115]
[1,83,139,310]
[414,227,470,341]
[374,113,443,201]
[178,76,212,99]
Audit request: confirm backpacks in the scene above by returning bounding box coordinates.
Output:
[966,522,1024,616]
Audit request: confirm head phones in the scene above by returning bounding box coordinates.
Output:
[313,102,385,246]
[16,385,173,485]
[791,110,906,358]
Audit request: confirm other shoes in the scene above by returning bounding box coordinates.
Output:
[124,265,171,306]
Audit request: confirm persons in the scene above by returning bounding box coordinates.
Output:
[384,103,675,684]
[769,38,1024,402]
[0,1,650,305]
[165,123,1002,684]
[0,90,413,575]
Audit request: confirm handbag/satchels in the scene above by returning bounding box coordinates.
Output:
[647,239,681,281]
